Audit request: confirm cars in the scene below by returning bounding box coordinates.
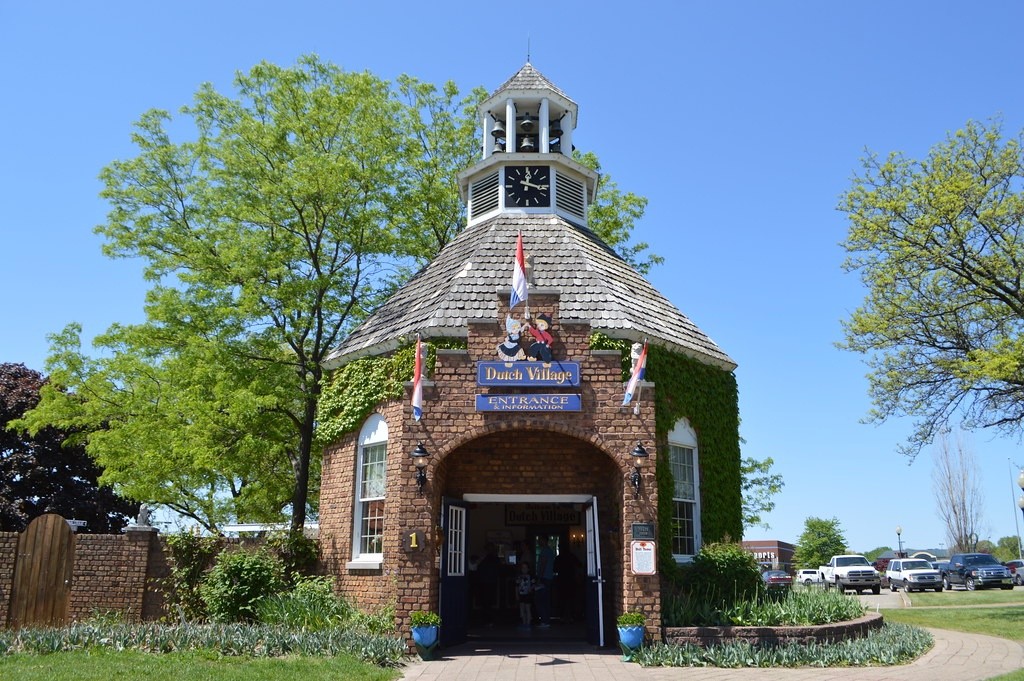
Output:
[795,567,822,587]
[1006,559,1024,586]
[760,570,792,589]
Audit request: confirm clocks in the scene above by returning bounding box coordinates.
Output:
[504,165,551,208]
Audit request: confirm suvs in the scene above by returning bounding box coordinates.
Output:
[886,559,943,594]
[818,554,883,594]
[942,552,1016,592]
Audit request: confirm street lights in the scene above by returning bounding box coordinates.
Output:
[895,523,904,555]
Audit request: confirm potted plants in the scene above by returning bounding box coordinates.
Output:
[409,608,442,648]
[617,612,647,649]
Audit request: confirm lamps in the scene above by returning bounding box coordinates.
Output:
[490,112,563,154]
[630,440,650,498]
[410,441,430,496]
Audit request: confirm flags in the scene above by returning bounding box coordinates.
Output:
[412,339,423,421]
[509,232,529,310]
[621,341,647,405]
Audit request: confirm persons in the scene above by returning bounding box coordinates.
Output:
[533,535,555,628]
[469,539,537,628]
